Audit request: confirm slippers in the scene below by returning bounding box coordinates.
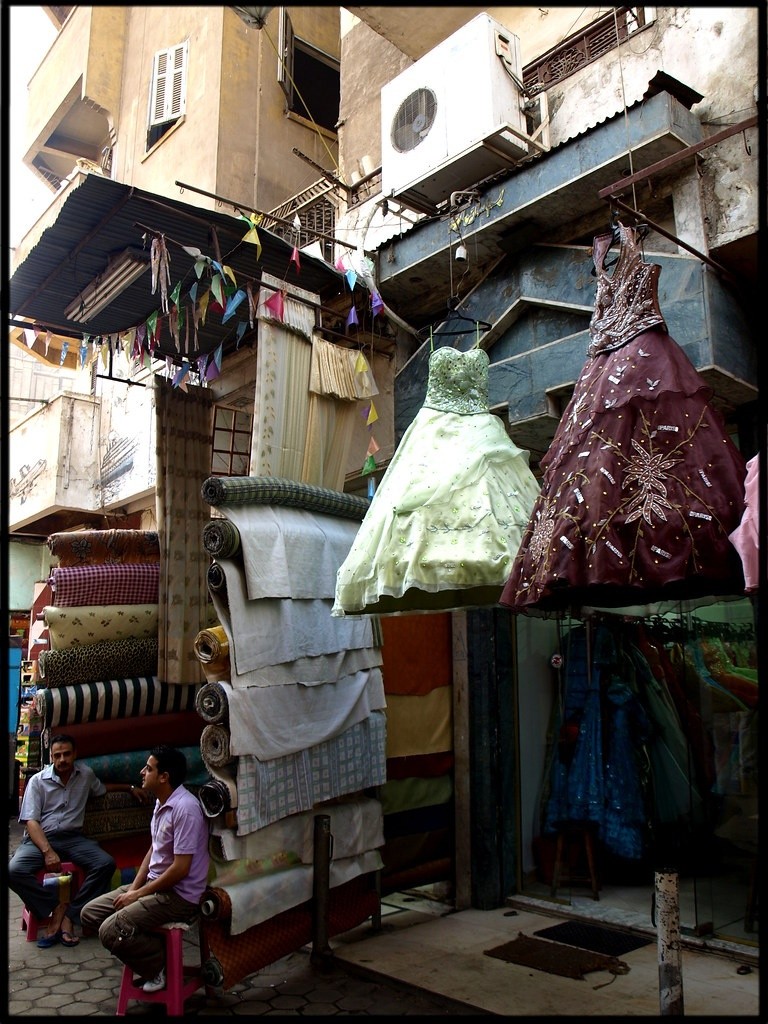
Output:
[59,929,80,946]
[37,930,61,948]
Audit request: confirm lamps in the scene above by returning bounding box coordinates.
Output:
[455,242,467,262]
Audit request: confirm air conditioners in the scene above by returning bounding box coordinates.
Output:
[380,13,528,215]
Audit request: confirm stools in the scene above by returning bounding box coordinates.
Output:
[116,916,215,1016]
[21,862,85,942]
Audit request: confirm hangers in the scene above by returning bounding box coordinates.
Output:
[564,614,754,651]
[415,298,493,338]
[590,210,652,278]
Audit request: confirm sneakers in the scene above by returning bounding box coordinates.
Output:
[143,967,167,991]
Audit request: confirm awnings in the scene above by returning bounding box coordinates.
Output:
[10,172,353,378]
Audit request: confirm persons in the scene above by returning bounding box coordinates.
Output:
[9,733,149,948]
[80,748,209,991]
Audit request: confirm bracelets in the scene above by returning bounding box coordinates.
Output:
[130,785,135,792]
